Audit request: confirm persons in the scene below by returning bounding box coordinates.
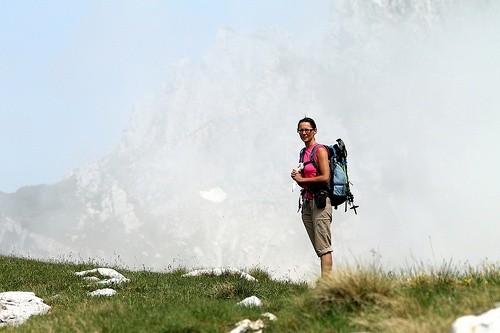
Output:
[291,118,334,278]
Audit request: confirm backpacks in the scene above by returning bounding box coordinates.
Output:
[300,139,350,207]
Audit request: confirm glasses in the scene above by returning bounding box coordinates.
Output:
[297,128,314,133]
